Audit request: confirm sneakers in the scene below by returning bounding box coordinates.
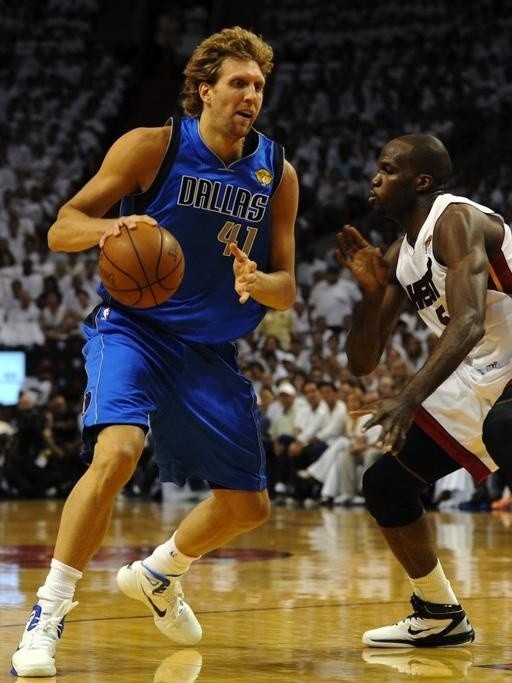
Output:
[269,471,366,509]
[422,485,511,513]
[10,604,68,677]
[116,558,203,645]
[362,591,475,649]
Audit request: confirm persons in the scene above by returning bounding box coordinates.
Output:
[10,24,302,678]
[332,128,512,652]
[2,2,512,512]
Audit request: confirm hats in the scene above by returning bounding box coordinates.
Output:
[276,380,295,398]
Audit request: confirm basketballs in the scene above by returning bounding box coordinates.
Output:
[101,220,185,308]
